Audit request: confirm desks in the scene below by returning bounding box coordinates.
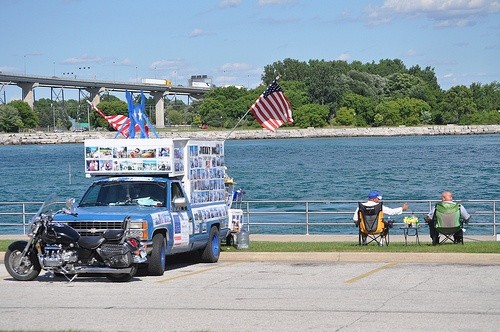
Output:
[399,225,421,246]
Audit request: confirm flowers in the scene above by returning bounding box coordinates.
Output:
[403,216,418,224]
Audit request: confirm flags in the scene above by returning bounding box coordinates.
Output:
[249,79,294,132]
[86,99,150,139]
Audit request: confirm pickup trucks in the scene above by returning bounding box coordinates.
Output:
[49,176,233,276]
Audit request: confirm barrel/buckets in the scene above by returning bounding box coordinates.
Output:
[238,230,249,249]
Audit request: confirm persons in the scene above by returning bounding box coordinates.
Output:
[424,191,471,246]
[353,191,408,246]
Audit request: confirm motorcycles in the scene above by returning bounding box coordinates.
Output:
[4,193,148,284]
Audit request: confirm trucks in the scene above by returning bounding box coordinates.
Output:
[141,79,171,86]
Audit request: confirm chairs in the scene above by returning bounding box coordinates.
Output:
[355,201,392,248]
[425,200,466,245]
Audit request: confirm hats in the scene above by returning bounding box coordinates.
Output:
[368,192,382,199]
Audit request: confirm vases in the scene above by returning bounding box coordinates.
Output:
[408,223,412,227]
